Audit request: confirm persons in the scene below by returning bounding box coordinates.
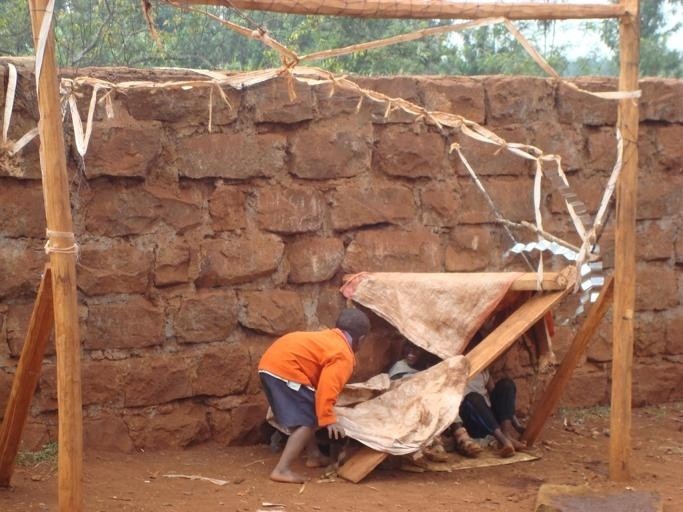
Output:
[257,307,371,484]
[459,332,526,456]
[389,341,484,461]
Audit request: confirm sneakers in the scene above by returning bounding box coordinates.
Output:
[423,426,485,463]
